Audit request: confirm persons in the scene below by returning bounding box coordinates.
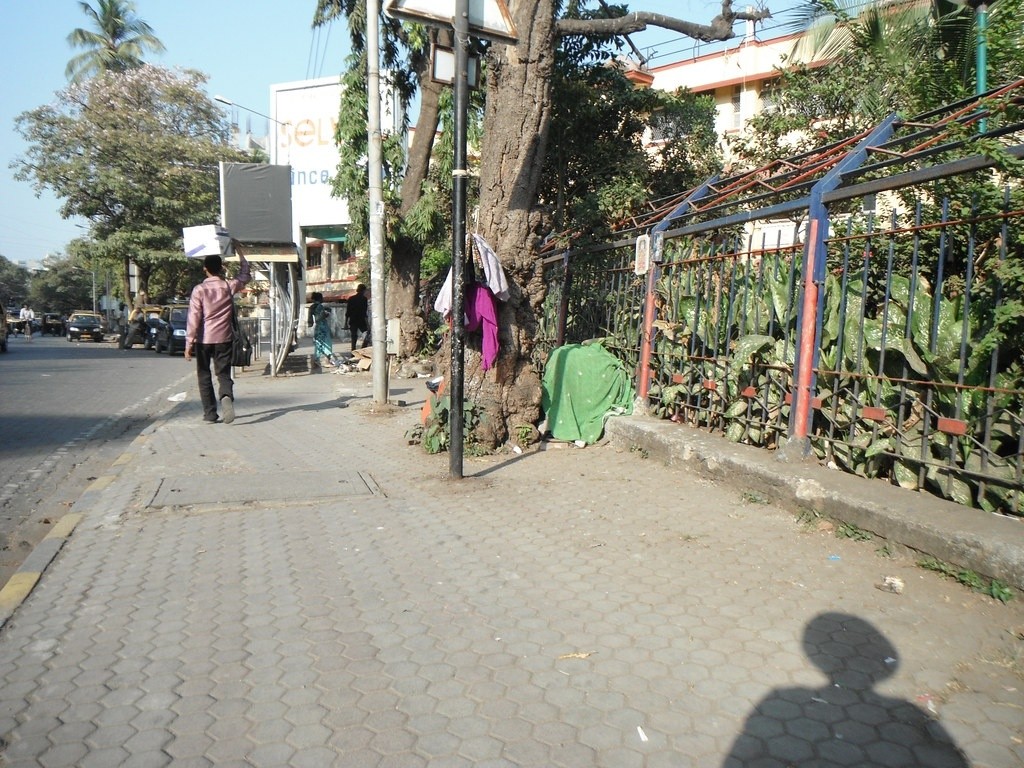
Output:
[307,292,332,362]
[19,302,35,338]
[124,307,143,348]
[62,312,69,336]
[184,238,251,424]
[117,302,126,349]
[344,284,371,357]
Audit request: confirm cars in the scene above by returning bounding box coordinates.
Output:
[66,315,102,342]
[68,310,105,340]
[128,305,163,350]
[32,321,40,331]
[0,303,9,350]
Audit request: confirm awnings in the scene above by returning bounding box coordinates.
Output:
[239,290,269,293]
[306,289,370,302]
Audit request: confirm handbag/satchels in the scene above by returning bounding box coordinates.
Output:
[231,322,252,368]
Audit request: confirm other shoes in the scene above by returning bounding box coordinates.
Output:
[222,395,235,424]
[324,363,334,368]
[203,420,216,423]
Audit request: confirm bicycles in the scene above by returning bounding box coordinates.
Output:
[21,317,35,342]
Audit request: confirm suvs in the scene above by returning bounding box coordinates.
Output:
[156,300,196,355]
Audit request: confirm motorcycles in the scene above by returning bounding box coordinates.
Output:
[41,313,63,336]
[7,310,24,338]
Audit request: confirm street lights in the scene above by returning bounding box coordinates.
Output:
[72,266,95,315]
